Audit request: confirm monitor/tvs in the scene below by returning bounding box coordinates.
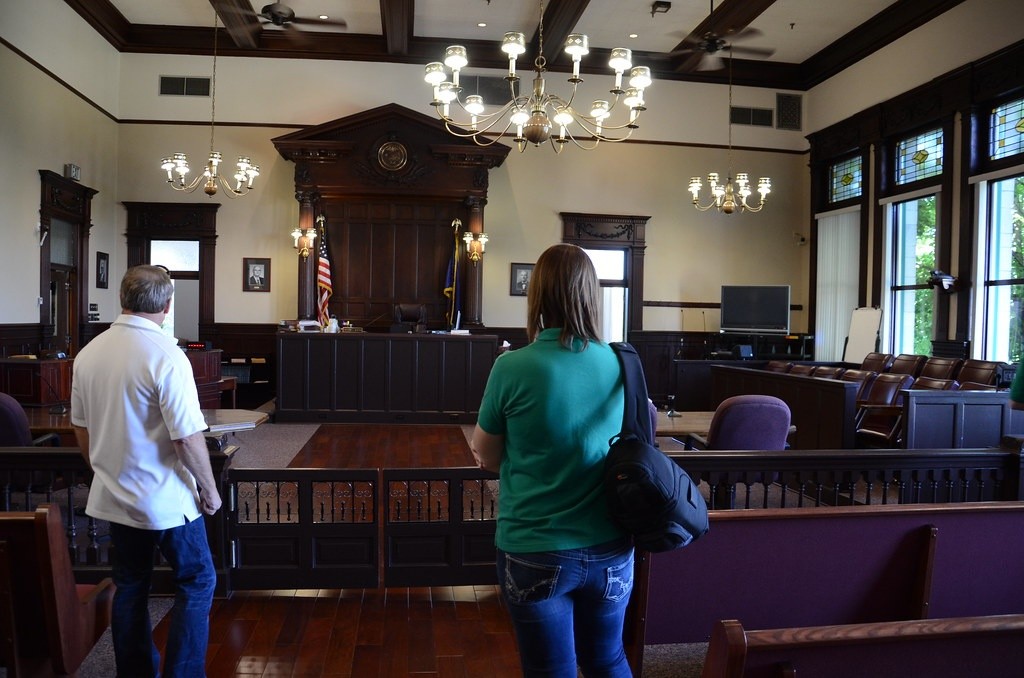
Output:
[721,285,791,336]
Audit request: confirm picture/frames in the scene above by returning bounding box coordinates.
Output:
[510,263,536,296]
[96,251,109,289]
[243,258,271,292]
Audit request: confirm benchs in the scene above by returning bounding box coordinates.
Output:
[0,502,117,678]
[622,500,1024,678]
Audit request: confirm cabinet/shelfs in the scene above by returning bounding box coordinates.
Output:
[672,332,815,412]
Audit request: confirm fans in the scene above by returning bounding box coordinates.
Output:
[208,0,347,45]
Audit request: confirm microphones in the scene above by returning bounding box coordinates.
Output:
[703,312,706,331]
[681,310,683,331]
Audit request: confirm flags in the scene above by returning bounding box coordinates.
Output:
[317,228,333,328]
[443,231,461,332]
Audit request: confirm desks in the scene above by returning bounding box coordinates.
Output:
[656,412,796,436]
[0,347,223,405]
[272,332,498,424]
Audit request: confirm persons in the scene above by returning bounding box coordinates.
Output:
[1008,357,1024,411]
[249,266,264,284]
[517,271,529,291]
[100,259,106,282]
[70,264,223,678]
[472,244,657,678]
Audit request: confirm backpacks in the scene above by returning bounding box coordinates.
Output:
[596,341,711,553]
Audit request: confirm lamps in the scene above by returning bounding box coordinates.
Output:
[687,43,771,217]
[290,227,317,262]
[424,0,652,155]
[160,13,260,199]
[463,232,488,267]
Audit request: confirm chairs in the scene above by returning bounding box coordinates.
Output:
[765,352,1000,485]
[683,394,790,512]
[390,304,427,332]
